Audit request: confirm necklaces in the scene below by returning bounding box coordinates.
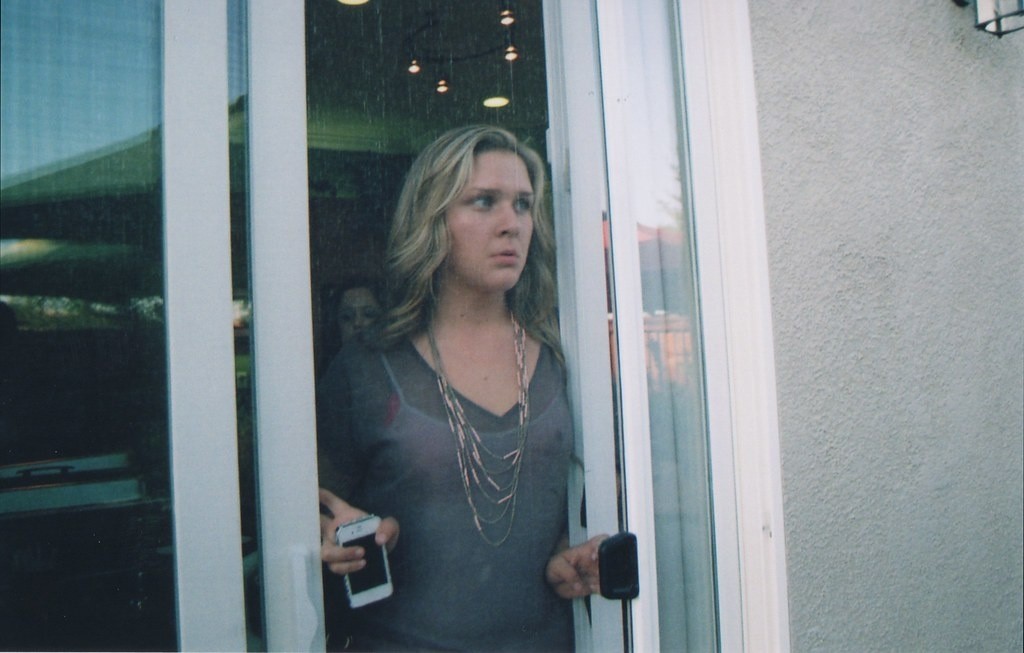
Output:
[425,311,529,547]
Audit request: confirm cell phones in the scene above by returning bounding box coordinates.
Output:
[335,515,393,609]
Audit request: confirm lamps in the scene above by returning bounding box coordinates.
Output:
[399,0,520,94]
[951,0,1024,37]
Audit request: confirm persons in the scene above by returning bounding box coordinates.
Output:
[327,278,387,362]
[316,125,609,653]
